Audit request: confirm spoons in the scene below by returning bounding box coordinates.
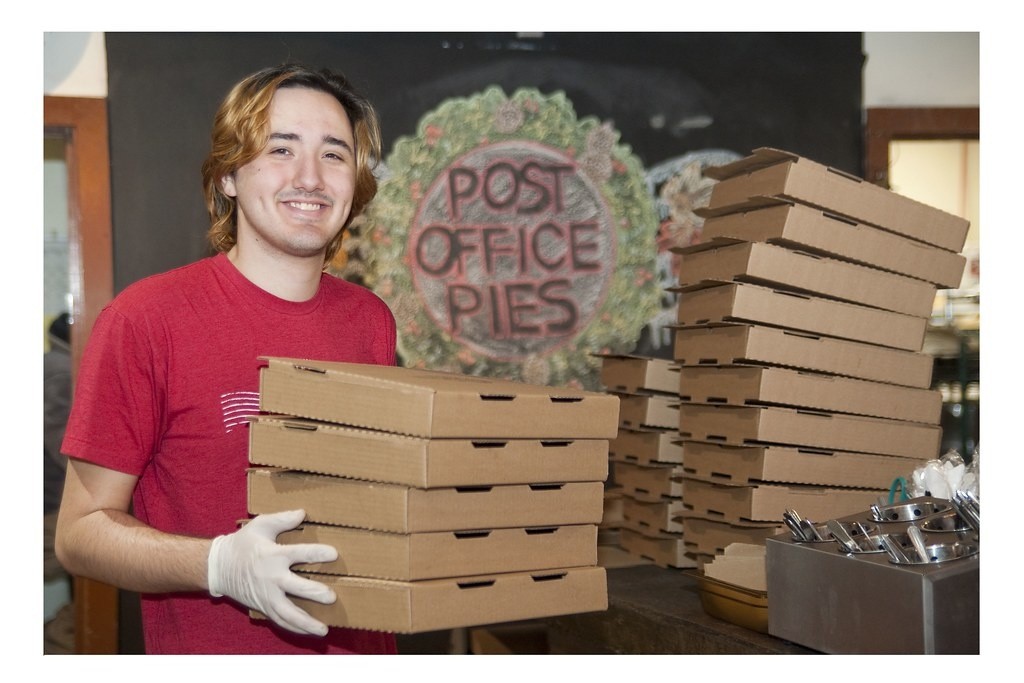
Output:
[905,455,980,501]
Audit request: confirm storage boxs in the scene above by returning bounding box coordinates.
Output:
[590,147,970,571]
[237,355,619,638]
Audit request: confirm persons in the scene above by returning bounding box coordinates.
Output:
[46,310,78,513]
[53,62,399,655]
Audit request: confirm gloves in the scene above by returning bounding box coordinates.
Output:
[206,509,338,638]
